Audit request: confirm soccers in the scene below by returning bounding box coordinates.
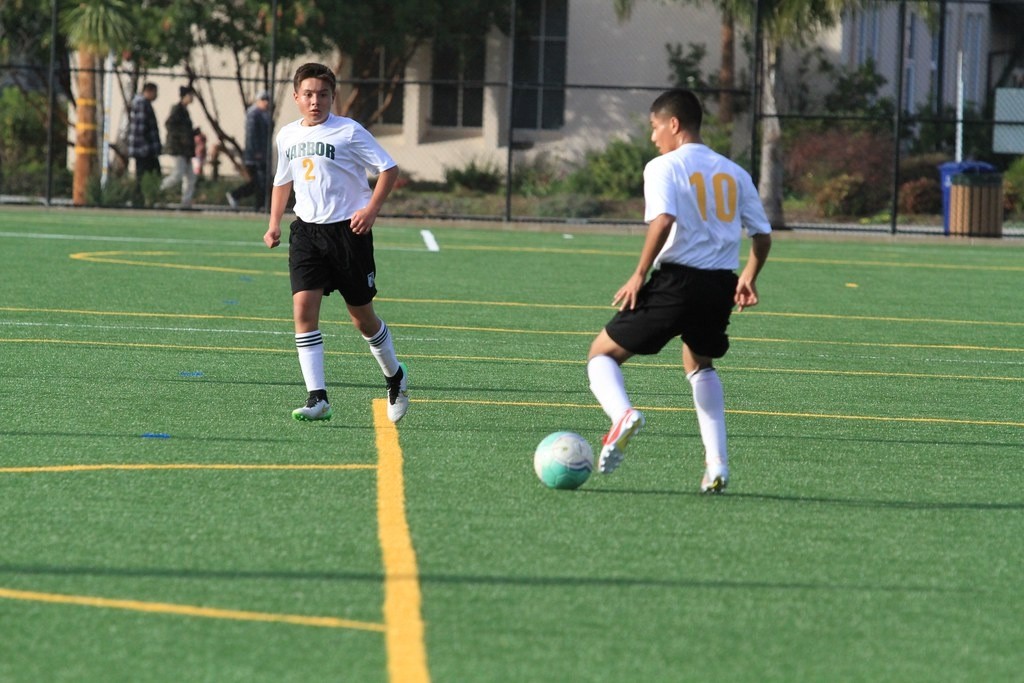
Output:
[533,431,595,490]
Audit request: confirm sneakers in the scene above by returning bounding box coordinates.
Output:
[598,408,646,475]
[385,362,409,422]
[291,396,332,422]
[698,456,729,496]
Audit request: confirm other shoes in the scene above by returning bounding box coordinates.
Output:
[250,206,265,213]
[225,191,236,207]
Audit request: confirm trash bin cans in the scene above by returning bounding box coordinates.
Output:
[938,161,1003,238]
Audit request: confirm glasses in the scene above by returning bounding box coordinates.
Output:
[259,96,270,102]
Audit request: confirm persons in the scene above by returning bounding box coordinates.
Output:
[262,64,409,423]
[125,83,164,209]
[586,89,772,494]
[226,96,270,213]
[159,87,201,211]
